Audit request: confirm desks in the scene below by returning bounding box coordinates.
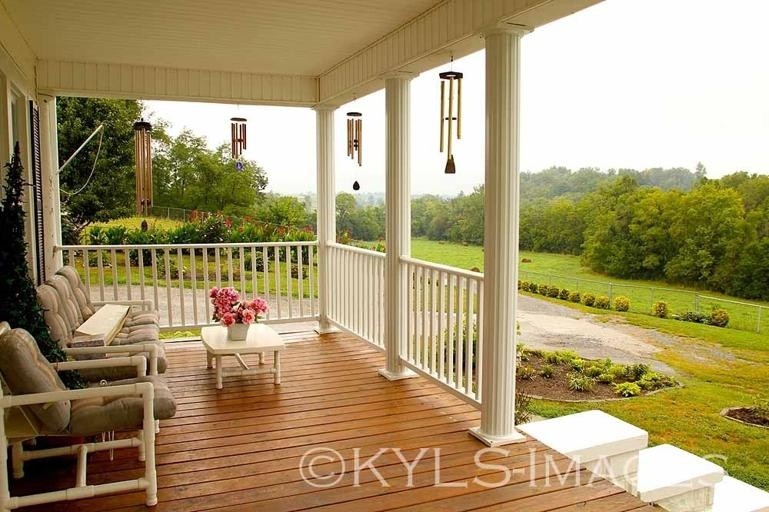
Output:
[201,325,285,390]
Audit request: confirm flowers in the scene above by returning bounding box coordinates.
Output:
[209,286,270,327]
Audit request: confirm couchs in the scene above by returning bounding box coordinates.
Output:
[36,266,167,381]
[0,322,177,512]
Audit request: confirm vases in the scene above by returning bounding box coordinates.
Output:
[225,324,250,341]
